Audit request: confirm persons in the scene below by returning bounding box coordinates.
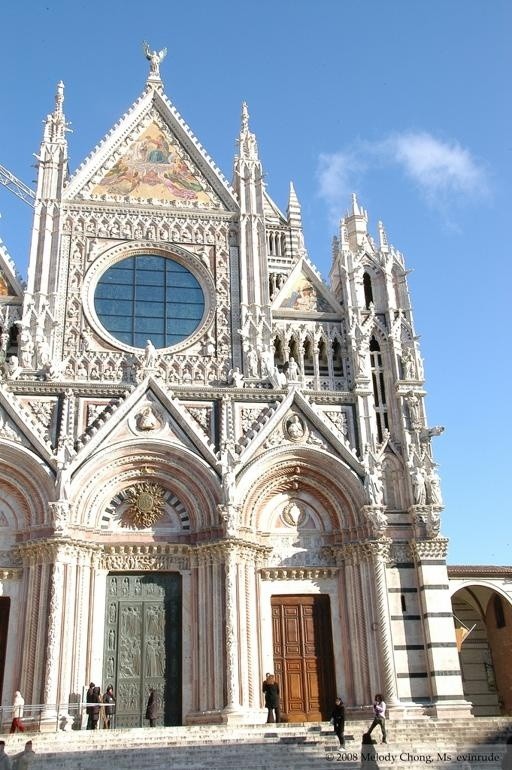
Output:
[63,220,231,383]
[35,337,51,369]
[91,687,100,729]
[102,683,116,730]
[45,365,62,382]
[363,471,384,507]
[288,415,304,440]
[54,462,74,503]
[241,100,250,133]
[226,366,247,388]
[0,741,9,770]
[103,576,181,679]
[143,45,168,76]
[263,675,281,723]
[354,351,366,379]
[400,346,418,379]
[149,135,170,163]
[263,344,275,377]
[262,673,270,708]
[11,740,35,770]
[55,79,65,112]
[20,335,34,368]
[145,688,159,727]
[135,135,150,164]
[245,344,260,377]
[86,683,95,729]
[405,389,420,426]
[221,465,237,503]
[9,691,25,735]
[137,407,158,431]
[330,698,346,749]
[285,357,300,382]
[410,467,428,505]
[365,694,387,743]
[424,468,443,505]
